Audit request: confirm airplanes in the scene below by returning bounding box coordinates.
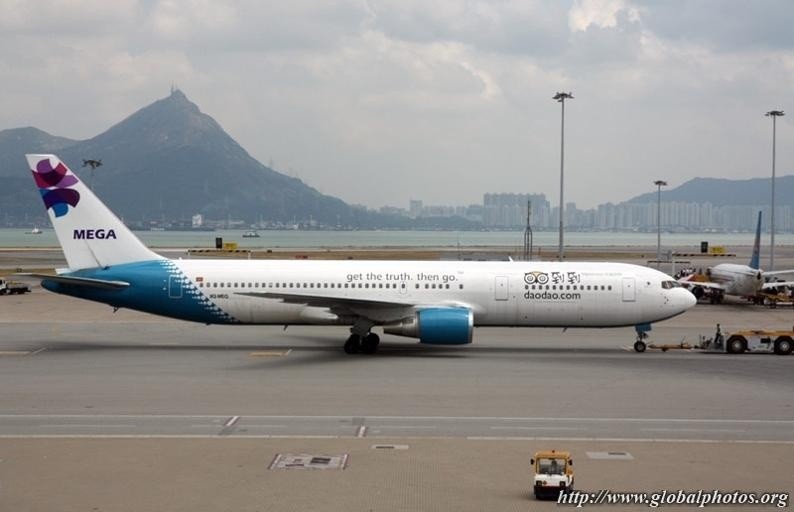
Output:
[11,153,697,354]
[677,210,794,304]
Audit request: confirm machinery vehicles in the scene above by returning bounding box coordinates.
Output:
[648,328,794,355]
[760,292,793,309]
[0,278,31,294]
[530,449,575,499]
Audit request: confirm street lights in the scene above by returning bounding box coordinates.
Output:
[764,109,784,271]
[653,179,668,261]
[551,90,574,261]
[81,157,102,191]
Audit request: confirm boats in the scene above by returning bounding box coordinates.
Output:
[24,227,42,234]
[242,230,260,238]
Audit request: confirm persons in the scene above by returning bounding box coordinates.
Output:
[762,285,794,308]
[549,461,561,474]
[676,266,712,279]
[714,323,724,342]
